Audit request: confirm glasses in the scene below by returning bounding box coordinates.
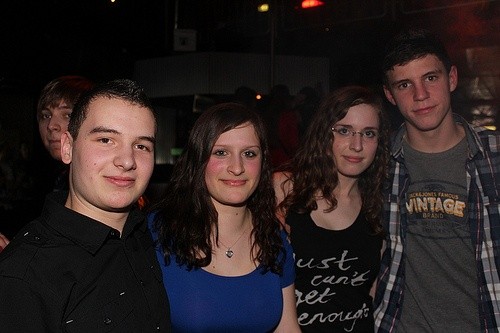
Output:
[332,127,380,140]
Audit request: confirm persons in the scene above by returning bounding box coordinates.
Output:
[0,75,150,251]
[147,103,302,333]
[0,79,295,332]
[275,86,391,333]
[370,30,500,333]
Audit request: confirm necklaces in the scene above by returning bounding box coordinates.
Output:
[210,230,246,259]
